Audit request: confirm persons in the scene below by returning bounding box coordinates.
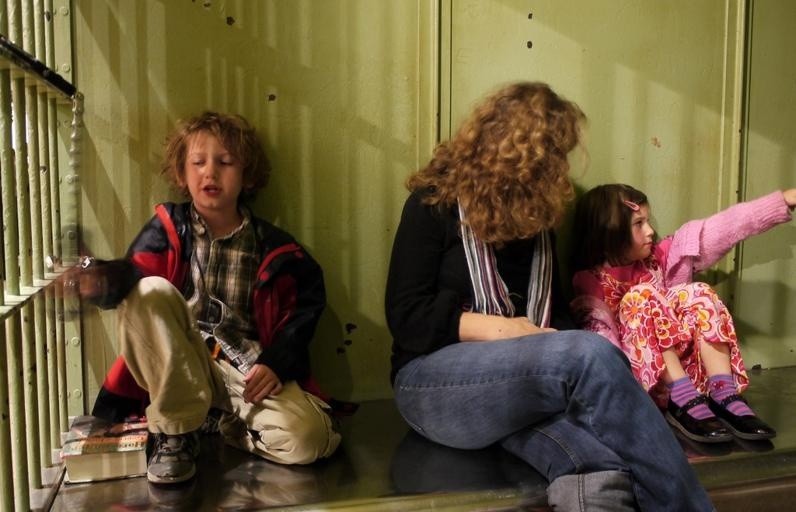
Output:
[383,81,720,512]
[568,183,796,445]
[65,109,343,484]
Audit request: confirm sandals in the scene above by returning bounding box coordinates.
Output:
[665,391,777,443]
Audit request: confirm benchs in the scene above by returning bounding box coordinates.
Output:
[53,364,796,511]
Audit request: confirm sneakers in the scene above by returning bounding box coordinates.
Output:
[146,433,200,484]
[201,408,219,434]
